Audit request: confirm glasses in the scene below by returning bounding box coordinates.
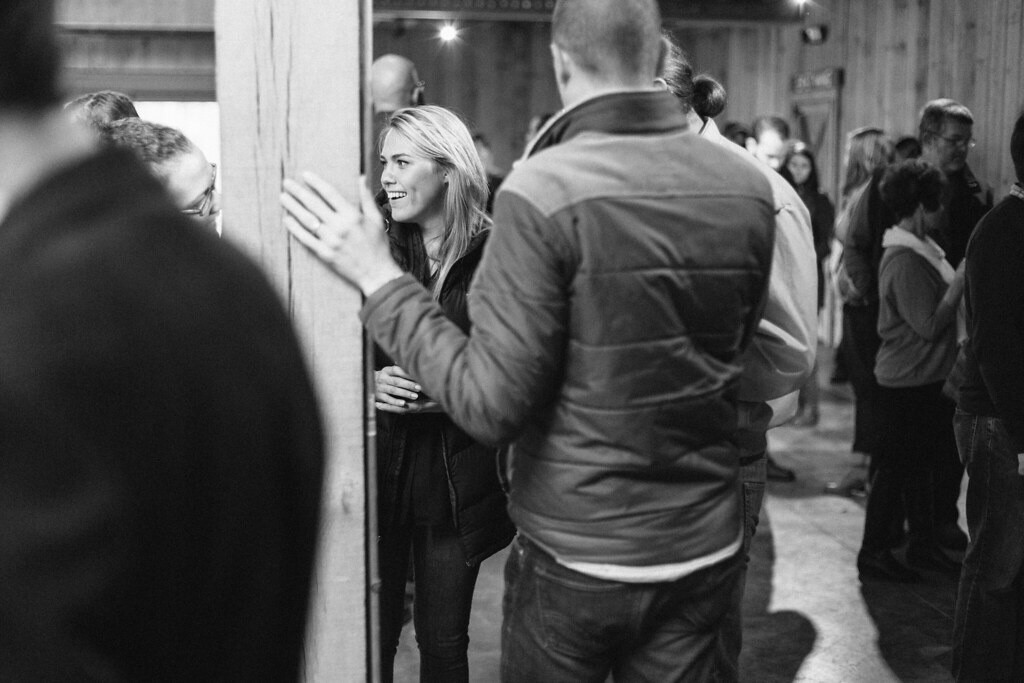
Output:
[180,161,220,216]
[926,128,977,149]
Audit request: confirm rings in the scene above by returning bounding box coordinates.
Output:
[312,221,322,235]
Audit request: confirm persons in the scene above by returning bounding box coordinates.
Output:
[277,1,836,683]
[825,98,1024,683]
[0,0,329,683]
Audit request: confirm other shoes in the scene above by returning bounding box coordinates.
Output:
[935,519,968,551]
[907,536,961,581]
[767,461,796,483]
[825,473,865,497]
[858,547,920,583]
